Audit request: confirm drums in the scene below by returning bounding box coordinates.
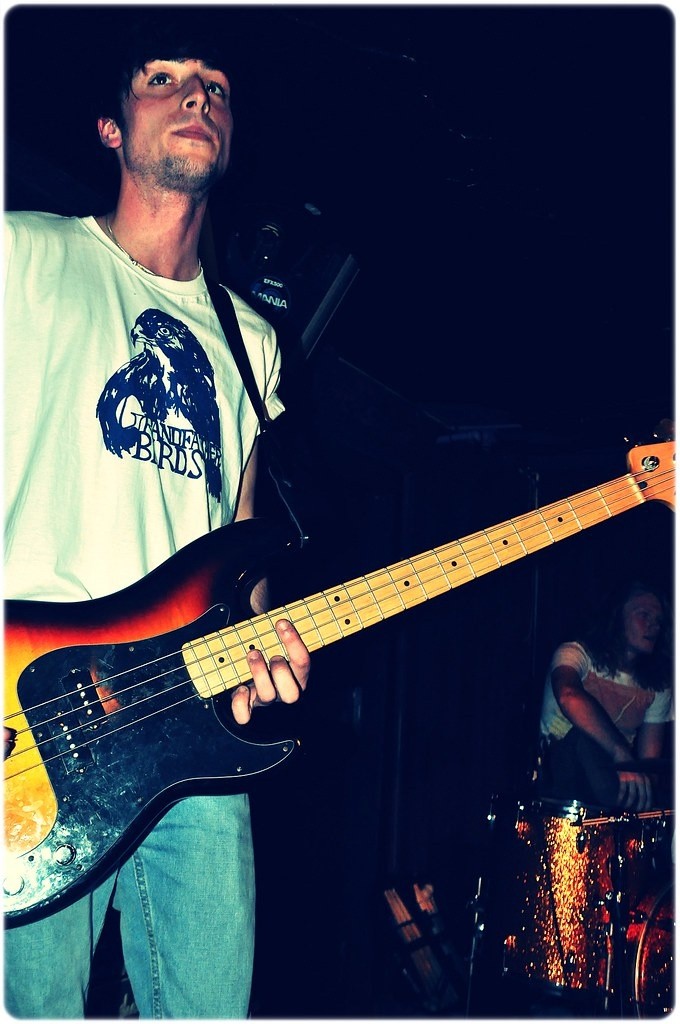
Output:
[497,792,661,997]
[612,869,671,1014]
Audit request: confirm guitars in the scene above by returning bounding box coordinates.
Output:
[2,416,673,925]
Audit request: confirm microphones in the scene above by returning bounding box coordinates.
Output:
[529,739,547,785]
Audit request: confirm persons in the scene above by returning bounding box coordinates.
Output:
[8,46,314,1017]
[540,578,672,810]
